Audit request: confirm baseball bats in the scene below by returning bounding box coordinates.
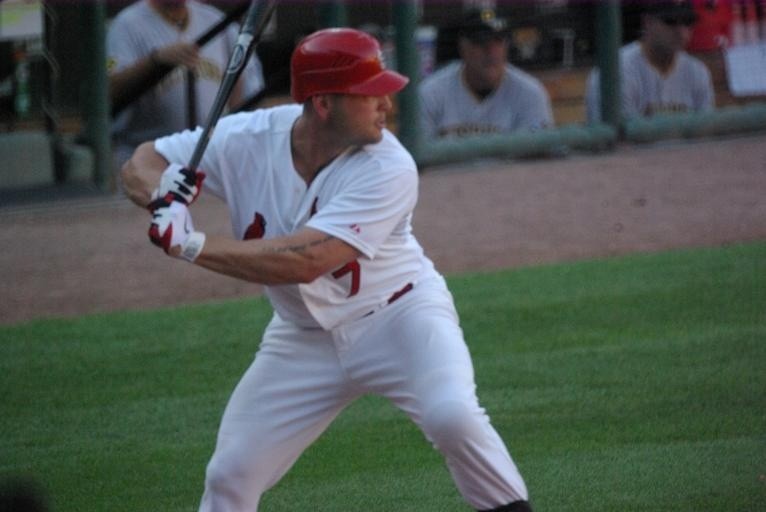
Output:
[188,0,278,172]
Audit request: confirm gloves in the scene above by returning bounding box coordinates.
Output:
[146,162,206,264]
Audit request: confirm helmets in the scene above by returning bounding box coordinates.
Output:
[291,26,410,104]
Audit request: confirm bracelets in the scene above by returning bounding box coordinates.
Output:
[146,48,164,84]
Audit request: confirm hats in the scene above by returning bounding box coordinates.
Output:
[641,1,698,22]
[459,8,506,44]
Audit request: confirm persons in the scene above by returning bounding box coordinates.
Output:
[104,0,266,195]
[585,0,766,124]
[120,26,532,512]
[417,10,554,145]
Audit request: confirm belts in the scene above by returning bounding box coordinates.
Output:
[363,282,412,317]
